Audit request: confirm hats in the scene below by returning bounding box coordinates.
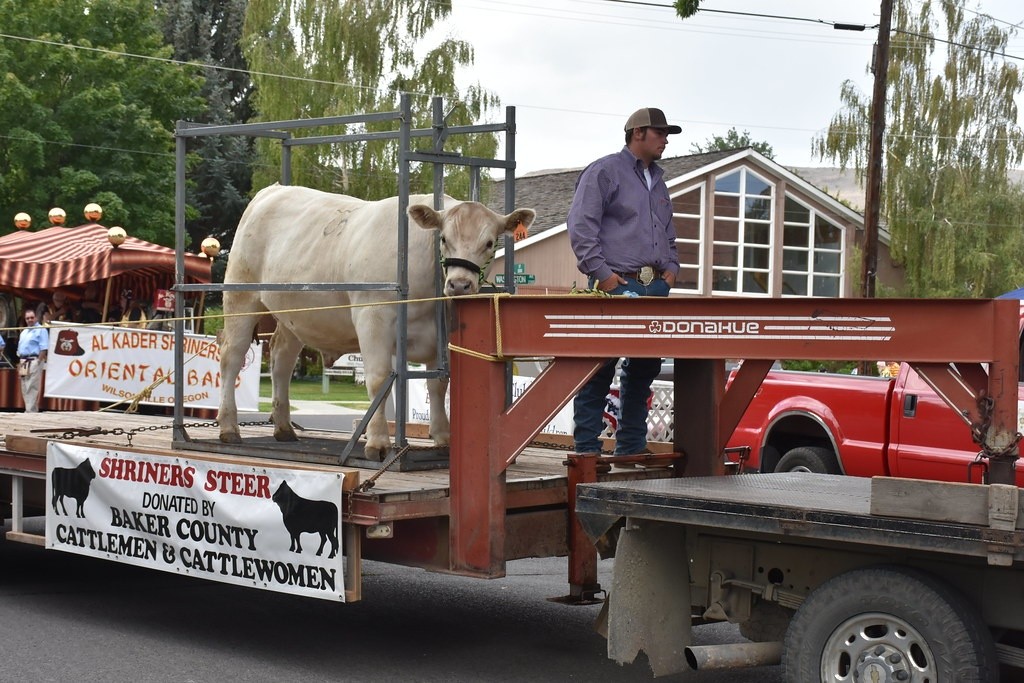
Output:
[624,108,682,134]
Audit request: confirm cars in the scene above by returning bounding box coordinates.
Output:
[392,357,676,443]
[617,357,786,378]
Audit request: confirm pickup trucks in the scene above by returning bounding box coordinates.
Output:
[722,361,1023,487]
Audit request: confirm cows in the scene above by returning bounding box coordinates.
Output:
[216,183,537,462]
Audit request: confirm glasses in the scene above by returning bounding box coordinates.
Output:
[25,317,35,320]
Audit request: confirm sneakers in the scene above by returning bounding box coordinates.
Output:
[595,462,611,472]
[614,448,674,468]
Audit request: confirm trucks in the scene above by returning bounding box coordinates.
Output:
[576,467,1024,683]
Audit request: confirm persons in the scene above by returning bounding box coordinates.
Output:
[567,107,682,469]
[818,361,900,378]
[0,285,149,412]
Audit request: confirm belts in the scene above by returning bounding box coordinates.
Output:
[20,355,39,358]
[612,266,664,287]
